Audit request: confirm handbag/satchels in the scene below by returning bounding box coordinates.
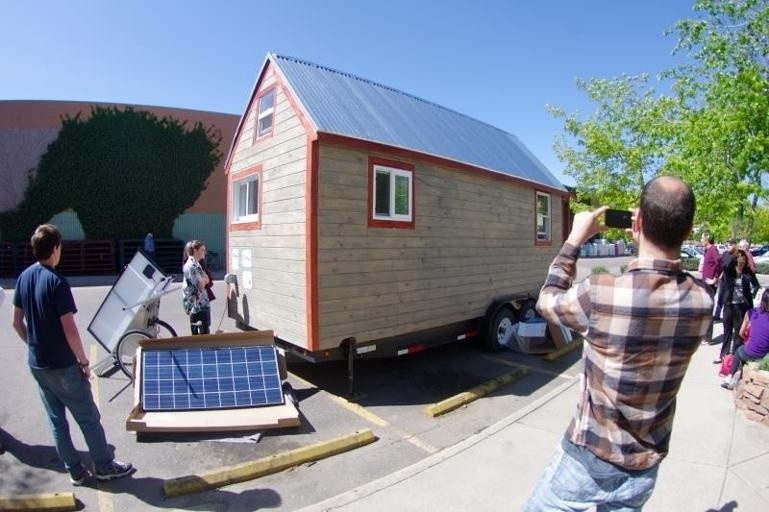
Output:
[742,319,751,343]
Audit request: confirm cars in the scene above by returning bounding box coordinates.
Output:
[681,243,769,260]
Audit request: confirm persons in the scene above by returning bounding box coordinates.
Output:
[698,231,722,346]
[144,230,156,257]
[10,221,135,487]
[712,240,739,324]
[713,249,761,364]
[179,239,217,335]
[721,288,769,391]
[737,238,757,273]
[522,173,716,512]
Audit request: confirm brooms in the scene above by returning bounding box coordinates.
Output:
[211,295,228,335]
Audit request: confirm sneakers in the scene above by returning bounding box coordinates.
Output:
[701,340,711,344]
[71,468,90,486]
[95,460,133,480]
[713,319,720,324]
[714,359,722,364]
[721,369,741,390]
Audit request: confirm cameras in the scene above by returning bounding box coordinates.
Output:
[605,209,633,229]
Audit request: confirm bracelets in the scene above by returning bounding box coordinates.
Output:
[80,359,89,368]
